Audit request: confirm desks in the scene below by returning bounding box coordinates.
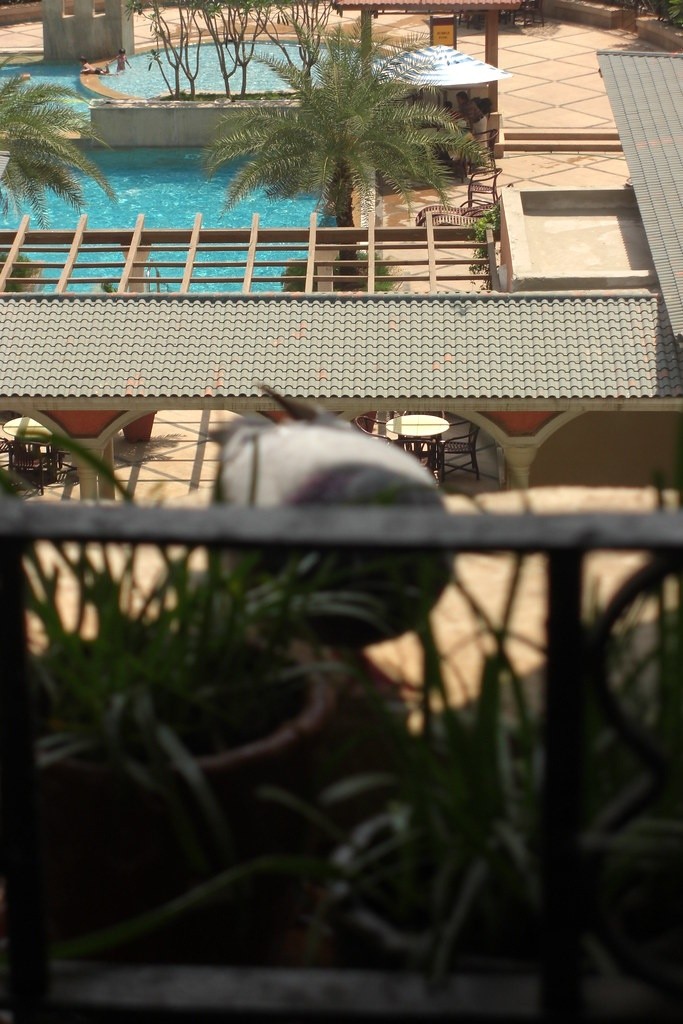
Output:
[386,415,450,436]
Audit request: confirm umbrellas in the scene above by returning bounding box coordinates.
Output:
[367,44,514,105]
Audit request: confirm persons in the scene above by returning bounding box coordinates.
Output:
[79,56,109,75]
[446,102,467,128]
[456,91,487,152]
[109,49,132,71]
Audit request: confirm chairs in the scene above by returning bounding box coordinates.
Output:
[416,182,514,226]
[355,415,392,444]
[513,0,544,28]
[442,419,481,482]
[394,439,438,476]
[468,168,503,208]
[460,129,499,183]
[7,440,54,495]
